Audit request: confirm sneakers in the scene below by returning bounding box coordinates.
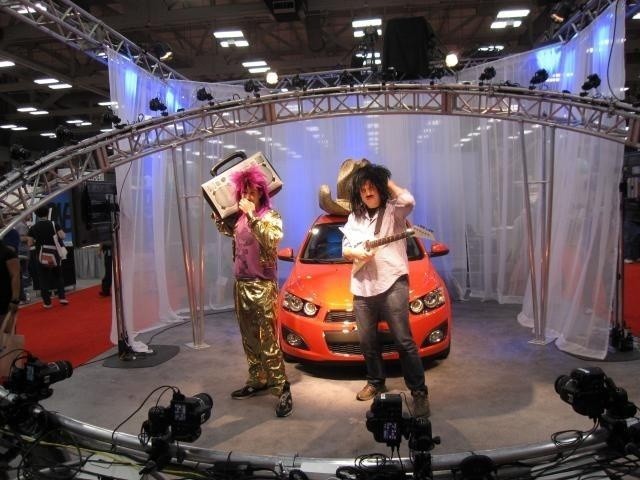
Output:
[231,385,268,399]
[357,383,385,400]
[275,393,293,417]
[412,391,430,417]
[19,299,69,308]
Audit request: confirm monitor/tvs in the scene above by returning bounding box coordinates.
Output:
[70,180,118,248]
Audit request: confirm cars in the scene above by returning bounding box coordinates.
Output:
[275,210,455,374]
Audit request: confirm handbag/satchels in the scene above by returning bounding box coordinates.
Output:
[0,326,25,377]
[53,233,68,260]
[37,244,61,268]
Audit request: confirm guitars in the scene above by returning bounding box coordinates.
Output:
[339,224,437,277]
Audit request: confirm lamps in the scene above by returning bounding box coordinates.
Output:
[9,125,72,165]
[429,65,602,97]
[550,1,585,25]
[101,77,260,130]
[143,39,174,62]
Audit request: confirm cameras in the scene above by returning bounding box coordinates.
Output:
[143,392,213,443]
[366,393,441,452]
[3,352,72,400]
[554,367,636,419]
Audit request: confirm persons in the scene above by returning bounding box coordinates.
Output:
[338,162,432,421]
[0,202,114,333]
[209,166,294,419]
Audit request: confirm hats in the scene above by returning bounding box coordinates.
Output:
[318,159,372,215]
